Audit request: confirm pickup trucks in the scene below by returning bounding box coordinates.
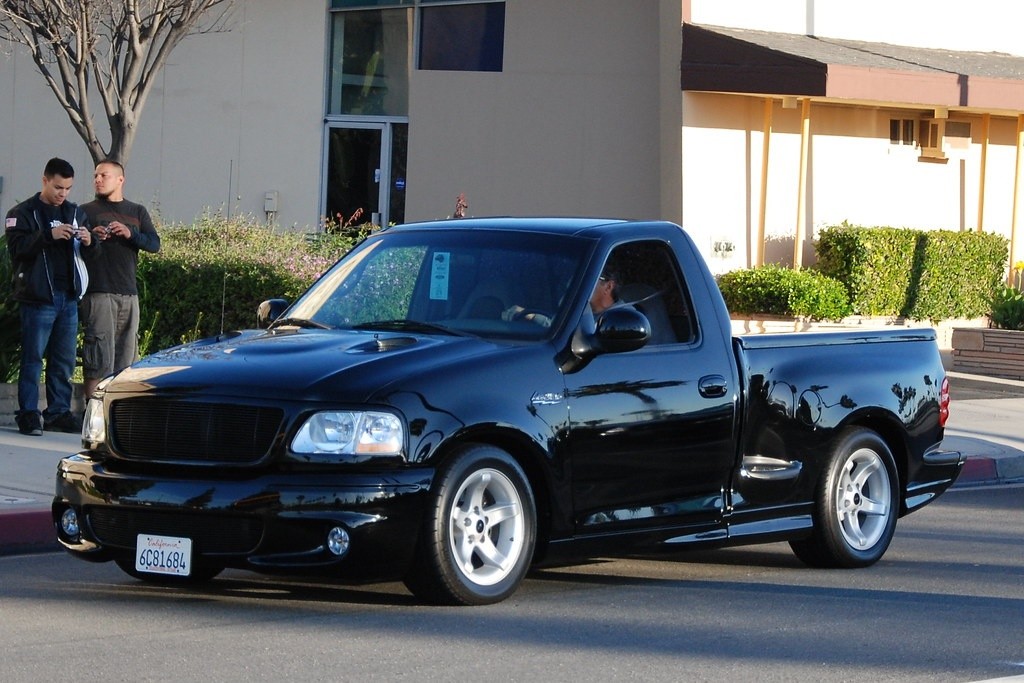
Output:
[50,218,965,600]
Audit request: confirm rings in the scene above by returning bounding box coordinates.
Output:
[507,309,512,313]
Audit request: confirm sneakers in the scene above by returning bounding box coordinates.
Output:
[15,408,43,436]
[41,408,84,433]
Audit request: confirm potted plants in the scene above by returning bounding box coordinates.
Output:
[949,280,1024,380]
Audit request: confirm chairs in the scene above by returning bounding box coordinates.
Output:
[461,244,524,316]
[611,260,676,342]
[530,257,570,302]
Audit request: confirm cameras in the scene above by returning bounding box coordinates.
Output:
[104,223,112,236]
[67,228,82,240]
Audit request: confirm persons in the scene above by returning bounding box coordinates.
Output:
[501,255,636,335]
[79,159,160,407]
[5,157,101,436]
